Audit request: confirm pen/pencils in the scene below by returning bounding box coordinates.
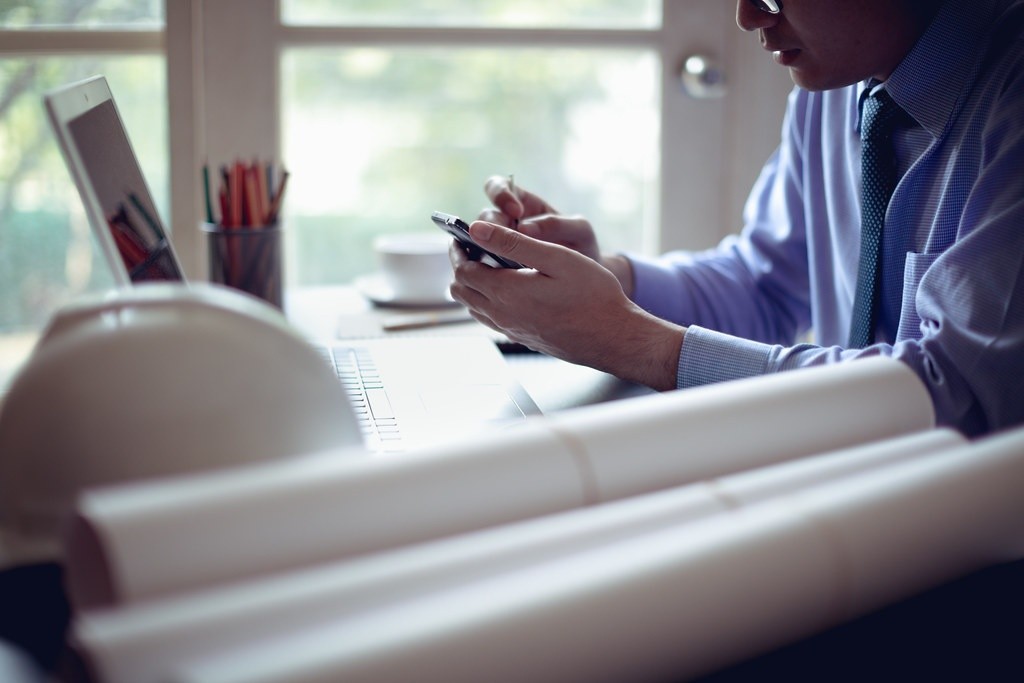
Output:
[199,154,289,225]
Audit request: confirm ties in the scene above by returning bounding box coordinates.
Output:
[846,88,904,352]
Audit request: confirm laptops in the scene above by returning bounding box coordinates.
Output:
[40,74,541,446]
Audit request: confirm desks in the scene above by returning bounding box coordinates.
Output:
[0,277,667,683]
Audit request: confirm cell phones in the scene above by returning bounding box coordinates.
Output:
[430,211,525,271]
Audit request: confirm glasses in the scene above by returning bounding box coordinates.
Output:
[751,1,795,18]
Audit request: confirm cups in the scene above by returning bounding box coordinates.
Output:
[372,229,458,300]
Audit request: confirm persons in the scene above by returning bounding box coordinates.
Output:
[446,0,1024,445]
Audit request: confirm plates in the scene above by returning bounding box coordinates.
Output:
[357,278,464,308]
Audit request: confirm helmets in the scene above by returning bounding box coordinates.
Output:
[0,281,366,620]
